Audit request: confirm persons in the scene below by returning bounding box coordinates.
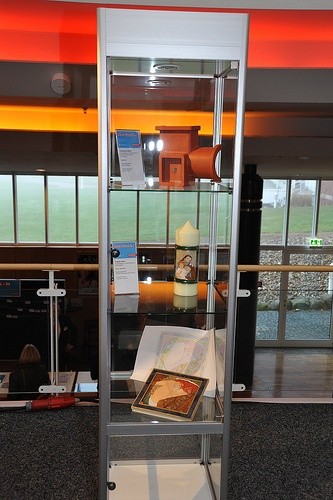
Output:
[42,303,78,372]
[7,344,51,400]
[176,254,196,280]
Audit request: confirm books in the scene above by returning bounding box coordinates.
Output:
[131,369,210,422]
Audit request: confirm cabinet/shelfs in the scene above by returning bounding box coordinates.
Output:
[96,8,249,500]
